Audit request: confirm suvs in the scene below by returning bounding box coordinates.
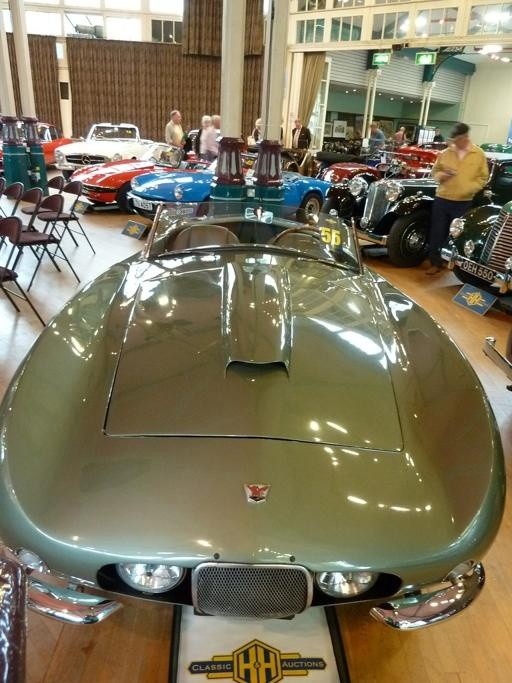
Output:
[337,151,512,269]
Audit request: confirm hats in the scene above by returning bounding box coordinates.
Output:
[447,123,469,141]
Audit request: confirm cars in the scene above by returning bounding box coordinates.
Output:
[244,144,315,179]
[184,128,224,151]
[0,122,72,172]
[438,200,512,302]
[315,142,449,185]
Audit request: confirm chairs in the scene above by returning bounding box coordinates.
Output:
[0,175,95,326]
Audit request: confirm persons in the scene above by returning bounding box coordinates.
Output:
[394,126,408,146]
[199,115,219,163]
[281,120,284,139]
[194,115,220,156]
[368,123,385,148]
[433,128,442,142]
[253,118,261,142]
[164,110,192,151]
[426,121,490,276]
[292,120,311,149]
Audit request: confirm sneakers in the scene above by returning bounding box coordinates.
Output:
[425,266,440,275]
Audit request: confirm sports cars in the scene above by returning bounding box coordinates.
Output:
[0,199,505,631]
[126,152,335,225]
[64,150,261,215]
[54,122,177,180]
